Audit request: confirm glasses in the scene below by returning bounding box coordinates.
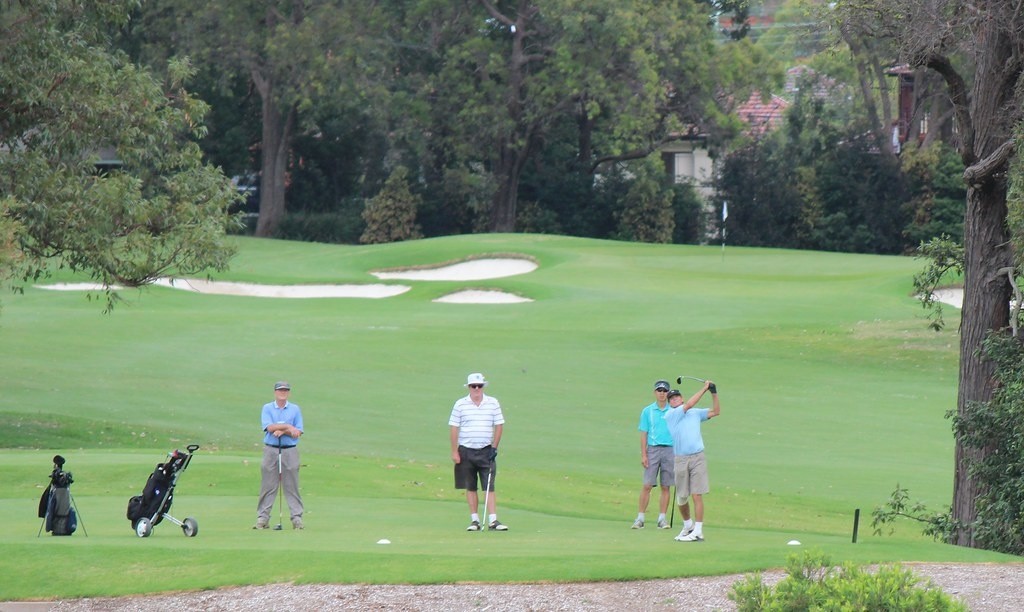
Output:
[469,384,484,389]
[657,388,668,392]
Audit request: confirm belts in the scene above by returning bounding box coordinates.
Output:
[266,444,296,449]
[658,445,668,447]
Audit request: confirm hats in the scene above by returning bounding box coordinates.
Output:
[668,389,681,398]
[468,373,487,387]
[654,381,670,392]
[274,382,290,390]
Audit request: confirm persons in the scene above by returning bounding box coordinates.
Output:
[449,373,509,531]
[665,381,721,541]
[631,381,679,531]
[253,381,305,530]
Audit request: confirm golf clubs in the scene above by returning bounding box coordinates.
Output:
[670,486,676,528]
[677,376,713,387]
[49,468,74,487]
[481,460,491,531]
[164,450,182,464]
[273,436,283,530]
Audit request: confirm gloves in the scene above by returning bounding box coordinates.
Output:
[488,448,497,462]
[709,383,716,393]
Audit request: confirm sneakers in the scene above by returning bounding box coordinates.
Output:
[674,529,693,539]
[467,521,482,532]
[679,531,704,542]
[488,521,508,531]
[658,520,670,529]
[253,522,269,530]
[631,519,644,529]
[292,518,304,530]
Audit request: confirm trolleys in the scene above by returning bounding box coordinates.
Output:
[127,444,200,538]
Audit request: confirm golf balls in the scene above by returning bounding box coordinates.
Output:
[511,25,516,33]
